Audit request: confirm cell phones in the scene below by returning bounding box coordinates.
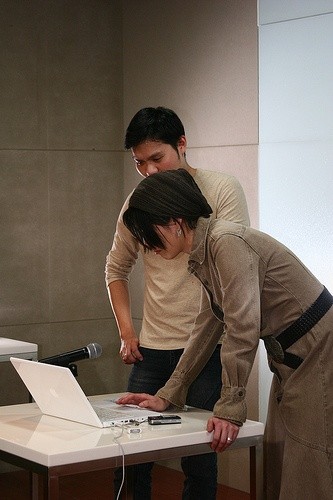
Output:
[148,415,181,425]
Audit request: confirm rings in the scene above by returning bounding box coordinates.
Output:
[227,437,234,441]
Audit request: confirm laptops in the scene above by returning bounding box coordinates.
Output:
[10,357,164,428]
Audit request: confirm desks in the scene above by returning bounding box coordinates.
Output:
[0,392,264,500]
[0,337,38,500]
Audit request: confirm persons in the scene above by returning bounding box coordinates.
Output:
[117,167,333,500]
[104,105,251,500]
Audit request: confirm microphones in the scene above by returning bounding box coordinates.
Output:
[36,342,103,366]
